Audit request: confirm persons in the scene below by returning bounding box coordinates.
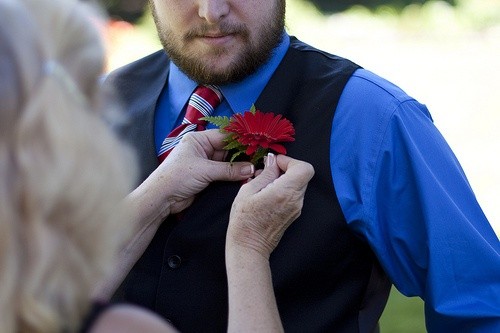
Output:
[97,0,500,333]
[0,0,314,333]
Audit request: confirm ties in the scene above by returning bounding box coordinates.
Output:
[158,83,224,162]
